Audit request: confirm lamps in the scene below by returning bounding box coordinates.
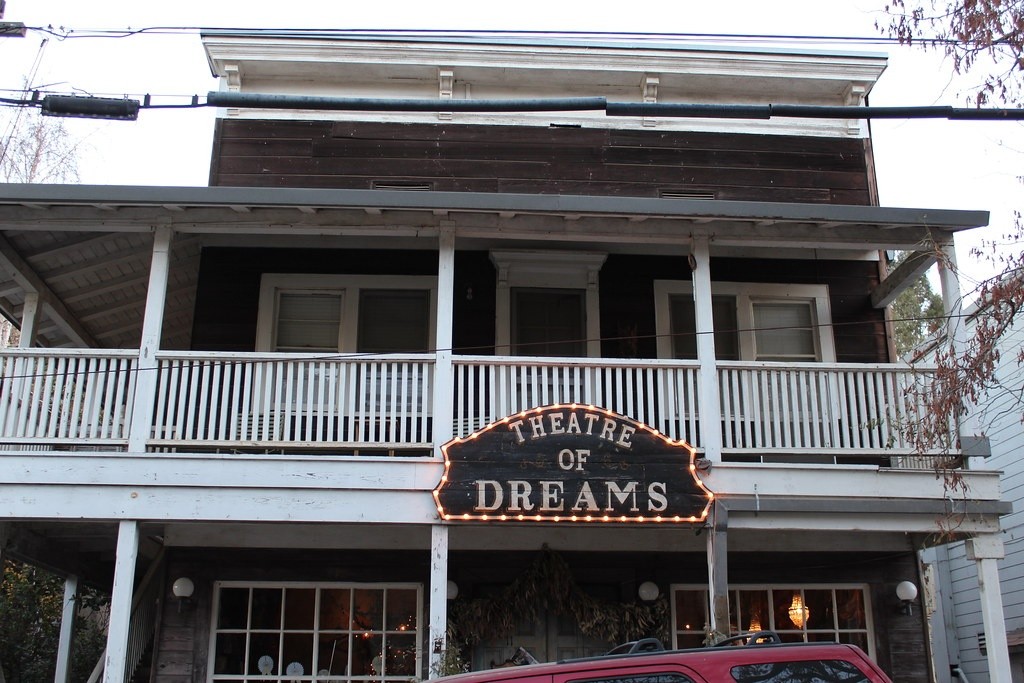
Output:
[447,580,458,612]
[173,577,195,614]
[897,582,917,616]
[639,583,659,614]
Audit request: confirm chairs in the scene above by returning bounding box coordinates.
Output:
[216,412,284,455]
[430,417,495,458]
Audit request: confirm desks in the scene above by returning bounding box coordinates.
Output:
[352,420,398,457]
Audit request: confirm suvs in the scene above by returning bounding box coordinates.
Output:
[420,631,893,683]
